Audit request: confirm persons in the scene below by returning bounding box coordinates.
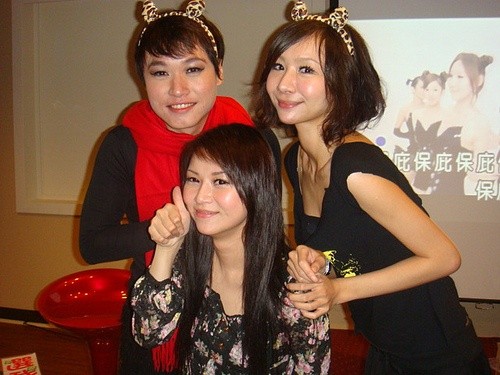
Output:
[251,0,493,375]
[393,54,500,196]
[79,0,283,375]
[131,121,333,375]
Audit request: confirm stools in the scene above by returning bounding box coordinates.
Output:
[35,268,132,374]
